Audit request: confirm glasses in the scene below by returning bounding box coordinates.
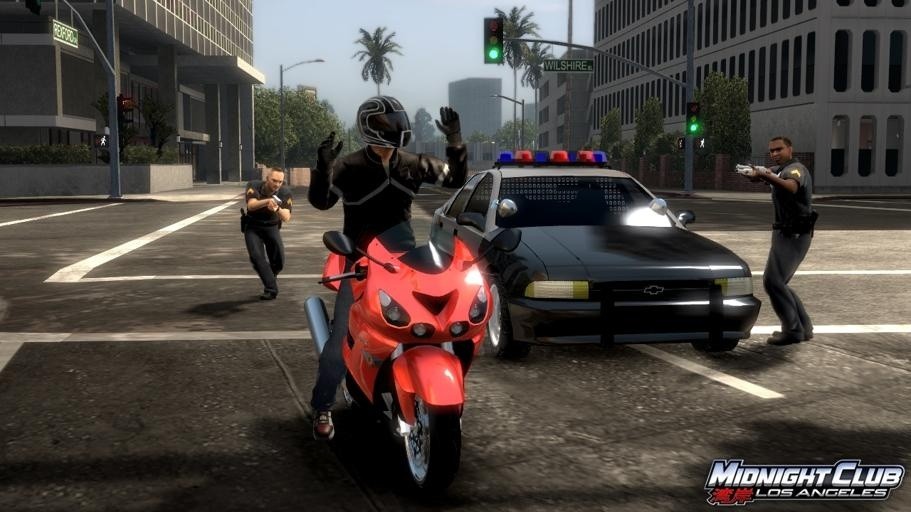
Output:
[769,148,785,155]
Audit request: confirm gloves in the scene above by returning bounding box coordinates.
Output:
[315,131,344,169]
[434,105,463,142]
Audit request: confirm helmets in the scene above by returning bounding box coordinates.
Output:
[356,95,415,151]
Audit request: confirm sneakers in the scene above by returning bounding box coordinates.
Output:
[310,406,337,444]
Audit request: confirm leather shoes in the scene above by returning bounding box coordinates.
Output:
[767,330,815,347]
[258,292,279,301]
